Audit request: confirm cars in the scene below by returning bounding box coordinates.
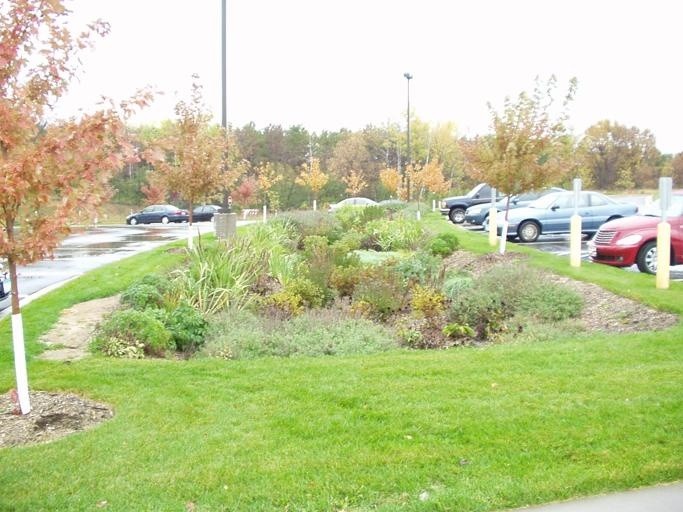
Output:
[125,204,223,225]
[438,182,683,276]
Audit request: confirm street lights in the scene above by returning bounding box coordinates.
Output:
[403,72,414,147]
